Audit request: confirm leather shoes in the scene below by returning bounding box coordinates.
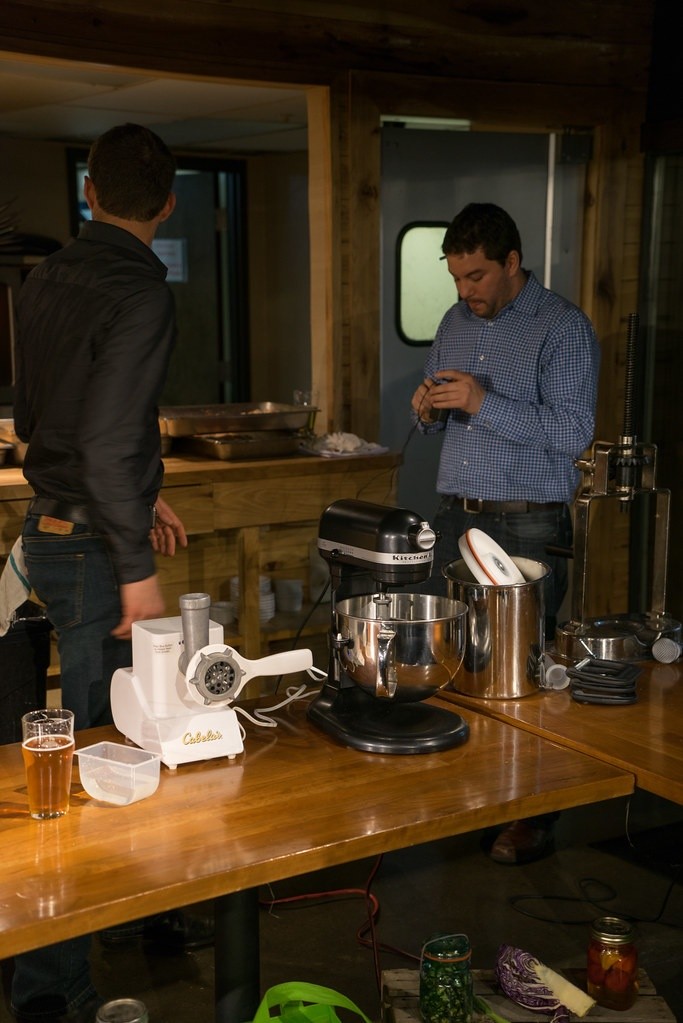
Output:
[493,807,560,863]
[103,915,220,950]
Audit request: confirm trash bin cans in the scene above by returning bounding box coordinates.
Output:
[0,599,52,744]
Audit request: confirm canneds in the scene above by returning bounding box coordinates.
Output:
[94,998,150,1023]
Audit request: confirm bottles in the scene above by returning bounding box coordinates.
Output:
[418,931,474,1023]
[95,998,149,1023]
[586,916,638,1012]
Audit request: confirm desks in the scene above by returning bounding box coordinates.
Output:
[0,682,636,1022]
[433,644,683,816]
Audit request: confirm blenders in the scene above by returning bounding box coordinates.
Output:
[304,498,470,755]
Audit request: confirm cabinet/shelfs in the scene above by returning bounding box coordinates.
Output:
[1,455,404,706]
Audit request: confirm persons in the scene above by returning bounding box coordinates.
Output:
[0,533,108,1023]
[410,203,597,864]
[6,122,215,955]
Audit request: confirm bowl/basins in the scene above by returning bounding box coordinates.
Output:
[329,594,468,705]
[278,579,304,613]
[209,601,234,626]
[229,575,275,626]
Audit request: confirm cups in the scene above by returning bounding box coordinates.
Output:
[293,388,320,438]
[21,709,76,820]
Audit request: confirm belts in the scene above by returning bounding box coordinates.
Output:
[29,497,156,528]
[441,495,565,514]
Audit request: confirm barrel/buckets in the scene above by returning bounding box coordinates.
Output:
[442,555,554,700]
